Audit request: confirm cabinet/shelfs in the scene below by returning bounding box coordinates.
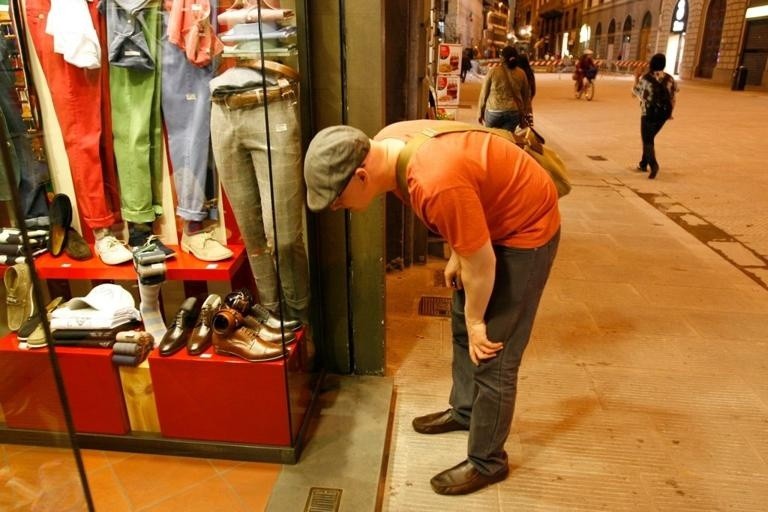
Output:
[447,79,458,100]
[449,52,460,71]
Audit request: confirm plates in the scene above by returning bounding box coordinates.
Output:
[0,212,314,449]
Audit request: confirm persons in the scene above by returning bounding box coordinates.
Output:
[573,49,598,100]
[476,47,533,136]
[302,117,563,497]
[516,53,537,131]
[630,53,680,180]
[460,48,473,83]
[210,78,317,358]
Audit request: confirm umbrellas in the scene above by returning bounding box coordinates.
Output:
[160,290,303,362]
[4,263,65,348]
[47,193,91,260]
[431,459,508,495]
[94,231,233,265]
[412,409,470,434]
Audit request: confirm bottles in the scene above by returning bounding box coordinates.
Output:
[303,125,370,213]
[68,283,136,310]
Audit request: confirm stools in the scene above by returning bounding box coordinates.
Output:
[211,84,296,110]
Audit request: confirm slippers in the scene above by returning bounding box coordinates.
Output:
[643,74,672,121]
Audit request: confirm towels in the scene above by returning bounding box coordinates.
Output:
[732,66,748,90]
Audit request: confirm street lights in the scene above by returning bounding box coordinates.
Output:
[519,106,531,130]
[513,125,571,197]
